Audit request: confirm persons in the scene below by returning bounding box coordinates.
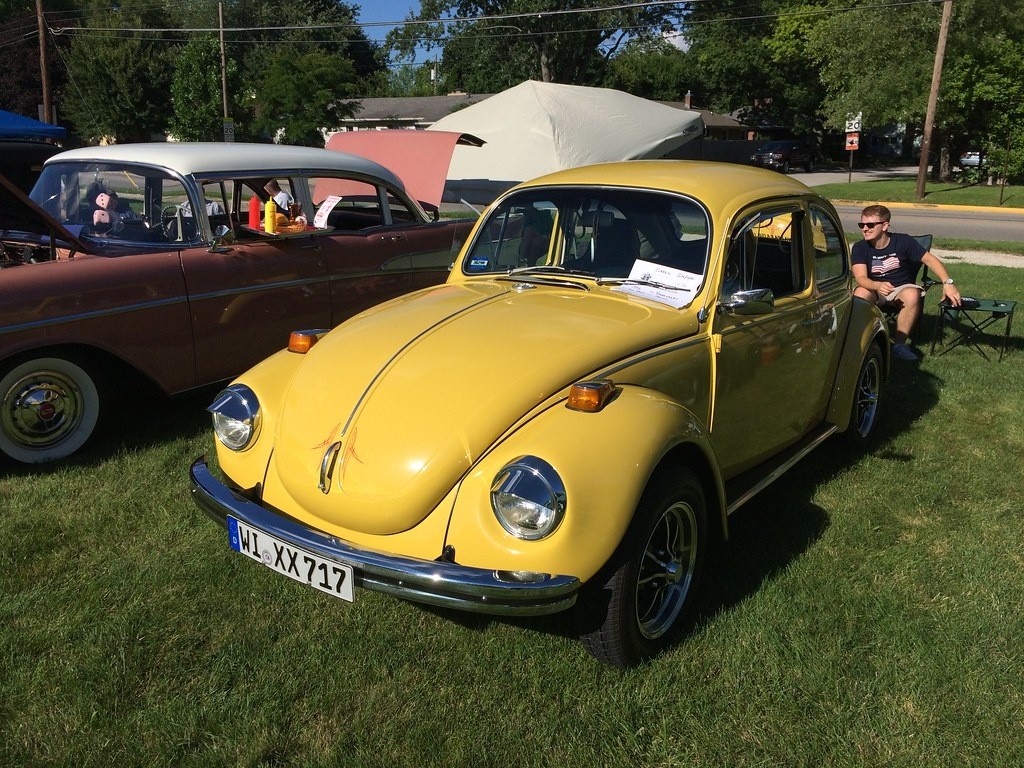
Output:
[175,186,225,218]
[850,205,961,346]
[265,179,291,210]
[82,181,138,220]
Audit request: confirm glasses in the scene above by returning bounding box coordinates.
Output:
[857,222,889,229]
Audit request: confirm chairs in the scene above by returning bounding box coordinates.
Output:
[555,217,793,303]
[908,234,933,345]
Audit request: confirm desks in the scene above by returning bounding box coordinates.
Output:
[930,296,1017,364]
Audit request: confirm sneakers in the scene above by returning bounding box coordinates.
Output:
[892,345,918,360]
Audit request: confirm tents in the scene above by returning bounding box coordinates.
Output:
[423,80,705,183]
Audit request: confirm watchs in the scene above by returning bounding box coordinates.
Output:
[943,278,954,284]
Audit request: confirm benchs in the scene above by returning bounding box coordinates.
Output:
[313,209,399,231]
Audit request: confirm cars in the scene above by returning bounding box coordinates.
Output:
[2,142,483,471]
[189,156,896,663]
